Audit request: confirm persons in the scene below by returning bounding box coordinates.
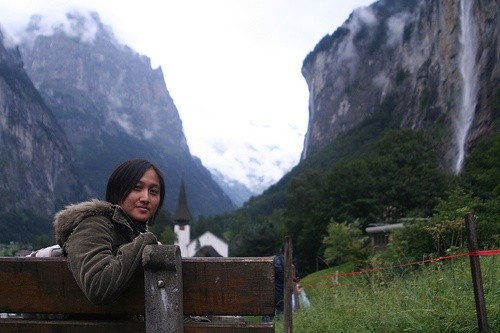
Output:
[262,241,285,324]
[53,159,167,323]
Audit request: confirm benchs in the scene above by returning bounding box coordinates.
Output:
[0,235,295,333]
